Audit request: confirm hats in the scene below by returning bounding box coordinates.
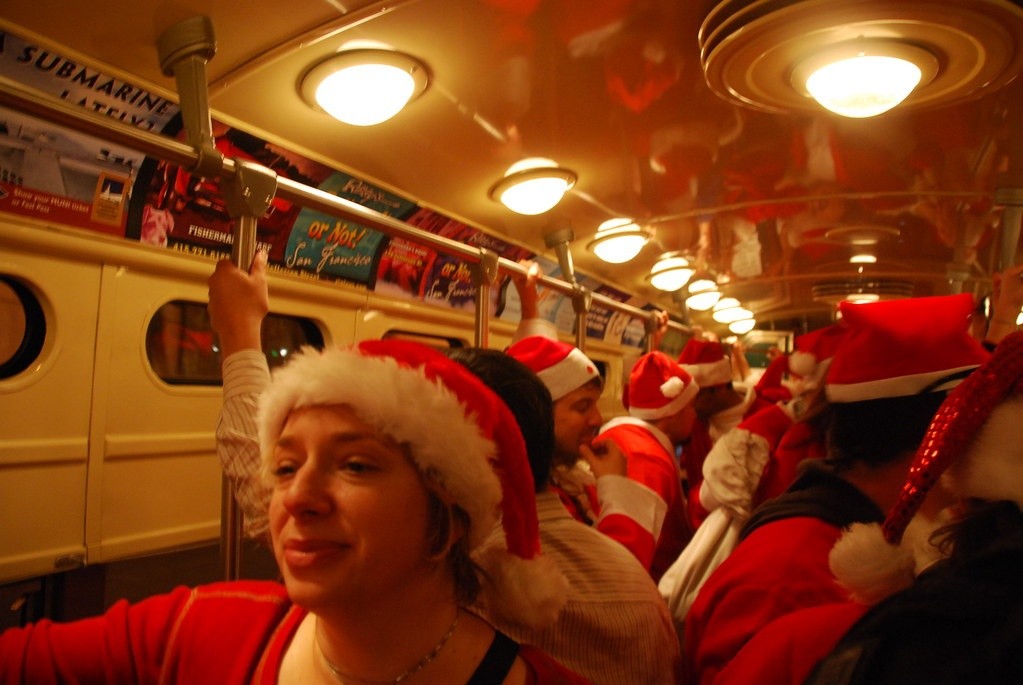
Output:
[679,336,733,389]
[504,336,601,404]
[628,350,700,421]
[789,322,842,384]
[831,328,1023,602]
[824,292,995,404]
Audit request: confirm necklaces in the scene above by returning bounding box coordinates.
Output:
[315,606,462,684]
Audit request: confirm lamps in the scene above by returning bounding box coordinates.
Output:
[713,298,755,334]
[646,257,696,293]
[812,281,915,304]
[685,279,724,312]
[299,47,429,128]
[697,0,1023,119]
[587,217,652,264]
[491,157,578,216]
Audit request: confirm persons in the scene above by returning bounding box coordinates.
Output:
[208,249,1023,685]
[0,338,596,685]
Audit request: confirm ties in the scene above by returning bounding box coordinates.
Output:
[258,339,569,629]
